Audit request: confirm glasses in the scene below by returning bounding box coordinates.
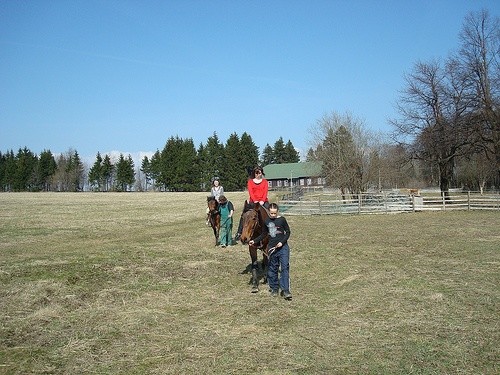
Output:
[271,213,276,214]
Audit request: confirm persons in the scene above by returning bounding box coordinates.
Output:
[206,194,235,248]
[206,179,224,225]
[235,166,270,241]
[248,202,292,298]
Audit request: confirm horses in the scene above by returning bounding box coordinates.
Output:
[206,196,221,246]
[240,200,273,293]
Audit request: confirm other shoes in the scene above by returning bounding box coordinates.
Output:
[272,289,279,297]
[282,290,293,299]
